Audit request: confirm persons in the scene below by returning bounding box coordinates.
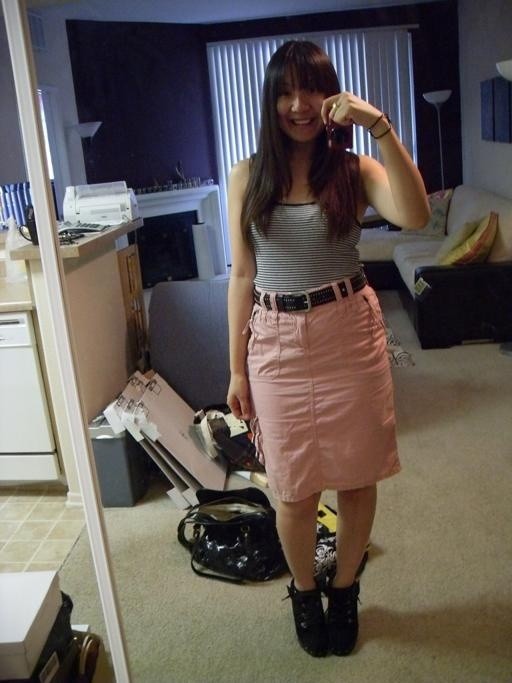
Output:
[224,39,434,657]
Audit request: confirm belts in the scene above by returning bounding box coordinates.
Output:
[252,273,368,313]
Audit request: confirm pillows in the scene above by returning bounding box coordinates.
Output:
[401,188,498,265]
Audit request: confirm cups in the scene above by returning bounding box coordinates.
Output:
[19,219,39,246]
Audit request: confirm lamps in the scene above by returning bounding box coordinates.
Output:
[422,91,452,190]
[496,60,512,83]
[73,122,103,184]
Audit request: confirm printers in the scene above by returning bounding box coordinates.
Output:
[62,181,139,227]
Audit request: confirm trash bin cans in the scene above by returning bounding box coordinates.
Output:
[88,410,149,507]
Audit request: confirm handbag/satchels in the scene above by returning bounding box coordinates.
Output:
[176,487,289,586]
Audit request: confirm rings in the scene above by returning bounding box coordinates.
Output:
[331,101,339,112]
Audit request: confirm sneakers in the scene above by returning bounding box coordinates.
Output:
[286,576,329,658]
[325,574,361,657]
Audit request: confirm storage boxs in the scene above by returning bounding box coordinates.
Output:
[0,571,78,683]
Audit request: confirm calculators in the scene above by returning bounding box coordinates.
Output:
[58,220,106,234]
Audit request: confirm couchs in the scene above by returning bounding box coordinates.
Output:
[357,186,512,350]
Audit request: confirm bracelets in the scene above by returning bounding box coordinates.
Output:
[365,111,395,140]
